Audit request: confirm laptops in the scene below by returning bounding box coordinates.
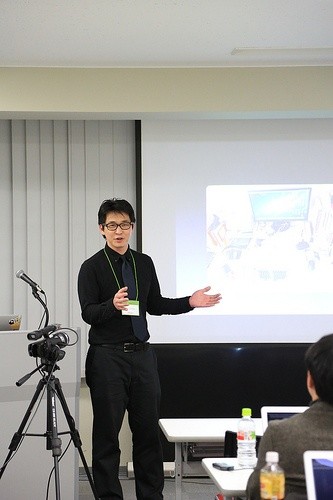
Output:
[303,450,333,500]
[261,406,310,435]
[0,314,22,331]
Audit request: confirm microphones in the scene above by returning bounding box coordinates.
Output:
[16,269,45,294]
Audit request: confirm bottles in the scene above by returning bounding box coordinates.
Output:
[259,451,285,500]
[236,408,257,469]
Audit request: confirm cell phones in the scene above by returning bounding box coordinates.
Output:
[213,463,234,471]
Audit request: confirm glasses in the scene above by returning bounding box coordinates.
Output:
[104,222,131,231]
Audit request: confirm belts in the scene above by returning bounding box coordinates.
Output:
[99,342,146,353]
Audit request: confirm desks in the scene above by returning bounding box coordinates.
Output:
[159,418,263,500]
[203,457,261,500]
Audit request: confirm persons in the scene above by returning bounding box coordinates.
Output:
[78,198,222,500]
[245,334,333,500]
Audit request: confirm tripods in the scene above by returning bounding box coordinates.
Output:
[0,359,99,500]
